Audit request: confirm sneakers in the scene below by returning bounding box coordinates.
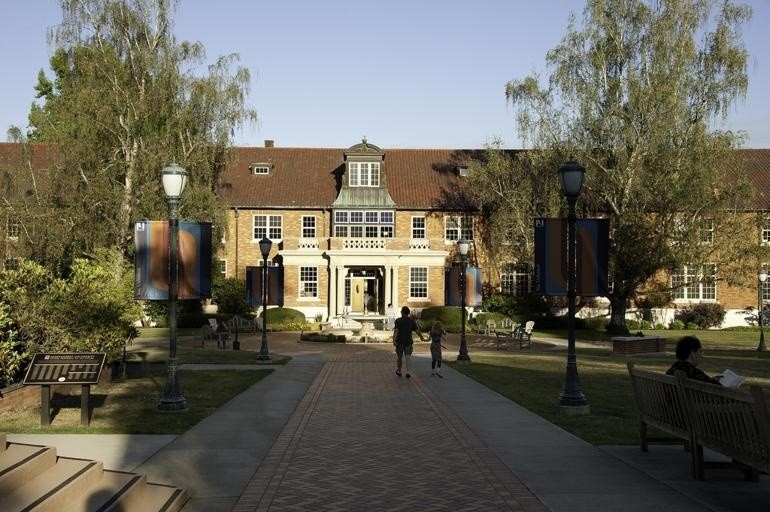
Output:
[432,370,444,378]
[395,370,412,379]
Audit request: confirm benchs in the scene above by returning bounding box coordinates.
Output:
[626,359,768,479]
[495,326,531,350]
[475,325,490,335]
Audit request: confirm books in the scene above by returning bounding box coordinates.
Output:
[718,369,746,391]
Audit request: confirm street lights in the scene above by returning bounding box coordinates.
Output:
[157,154,188,410]
[559,152,587,406]
[757,271,768,348]
[455,236,471,360]
[256,233,272,361]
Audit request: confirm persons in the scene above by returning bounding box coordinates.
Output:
[385,303,397,331]
[391,304,425,379]
[662,333,731,431]
[421,321,448,380]
